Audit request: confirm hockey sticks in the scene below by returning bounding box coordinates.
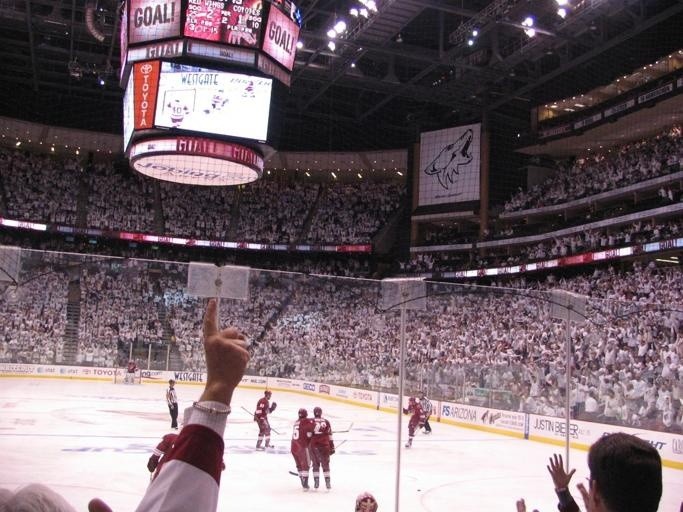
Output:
[241,407,287,435]
[331,422,353,433]
[289,440,344,476]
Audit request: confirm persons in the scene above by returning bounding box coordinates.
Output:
[242,77,255,97]
[289,408,314,490]
[516,432,664,512]
[251,390,277,450]
[400,398,423,448]
[309,408,337,488]
[0,482,78,512]
[0,125,683,434]
[167,94,191,126]
[202,87,228,114]
[88,497,115,512]
[133,297,252,512]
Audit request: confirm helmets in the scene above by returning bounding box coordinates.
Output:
[299,408,307,417]
[314,407,322,416]
[265,391,271,395]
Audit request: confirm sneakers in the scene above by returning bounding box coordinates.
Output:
[256,445,274,449]
